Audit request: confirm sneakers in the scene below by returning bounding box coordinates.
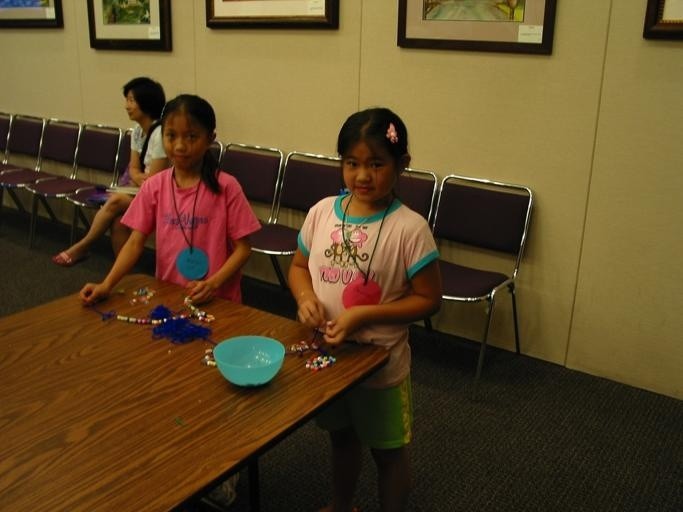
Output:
[208,471,240,508]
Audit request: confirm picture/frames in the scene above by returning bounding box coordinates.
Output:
[396,0,558,55]
[86,0,172,53]
[641,0,683,42]
[204,0,340,31]
[0,0,64,30]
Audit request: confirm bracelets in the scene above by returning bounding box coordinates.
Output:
[296,289,312,303]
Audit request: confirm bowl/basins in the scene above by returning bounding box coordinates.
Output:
[211,334,287,390]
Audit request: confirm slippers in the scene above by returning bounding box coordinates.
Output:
[50,251,90,267]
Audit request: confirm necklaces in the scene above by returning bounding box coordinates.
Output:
[344,201,383,240]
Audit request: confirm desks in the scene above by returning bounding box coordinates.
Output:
[0,272,389,510]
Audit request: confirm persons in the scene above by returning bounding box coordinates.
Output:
[284,104,444,511]
[49,77,165,268]
[74,91,261,511]
